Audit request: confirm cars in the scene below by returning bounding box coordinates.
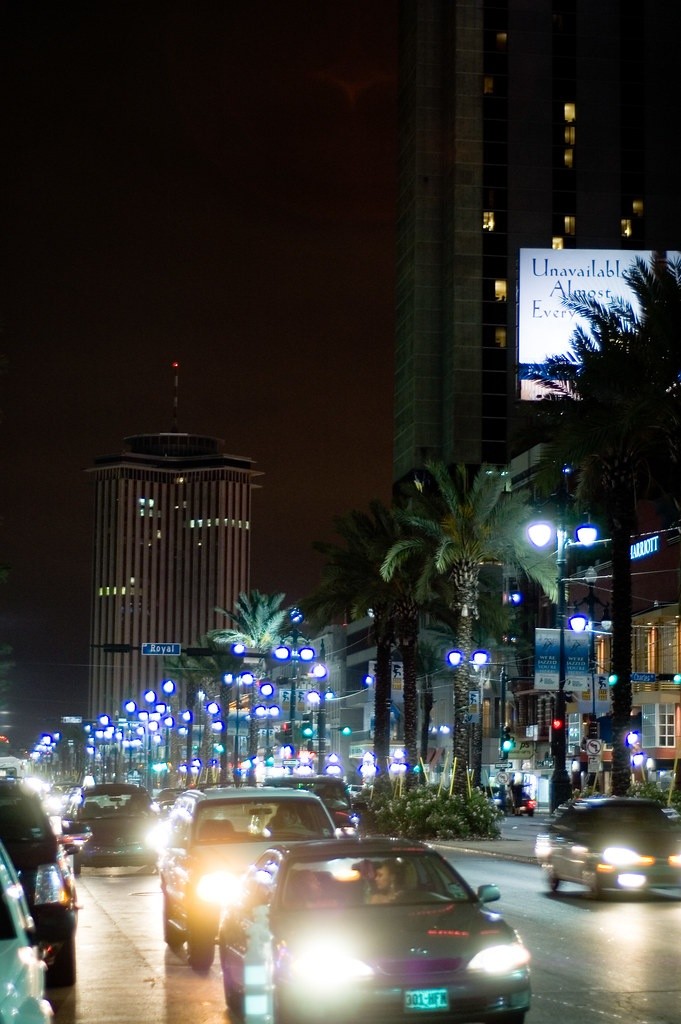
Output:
[215,834,533,1024]
[519,792,538,817]
[248,776,369,840]
[0,840,57,1024]
[531,796,681,901]
[40,782,196,876]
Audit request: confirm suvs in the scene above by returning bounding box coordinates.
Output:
[0,779,85,989]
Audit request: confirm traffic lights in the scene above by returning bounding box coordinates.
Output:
[498,725,513,760]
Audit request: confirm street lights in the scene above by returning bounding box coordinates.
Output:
[569,562,613,793]
[223,671,254,784]
[524,509,556,806]
[274,647,316,776]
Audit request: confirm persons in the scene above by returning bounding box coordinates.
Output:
[293,866,338,904]
[367,859,405,904]
[273,802,303,829]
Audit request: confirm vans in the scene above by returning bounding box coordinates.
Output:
[147,786,344,972]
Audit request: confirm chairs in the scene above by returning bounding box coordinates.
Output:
[360,858,418,902]
[85,802,100,808]
[199,819,233,839]
